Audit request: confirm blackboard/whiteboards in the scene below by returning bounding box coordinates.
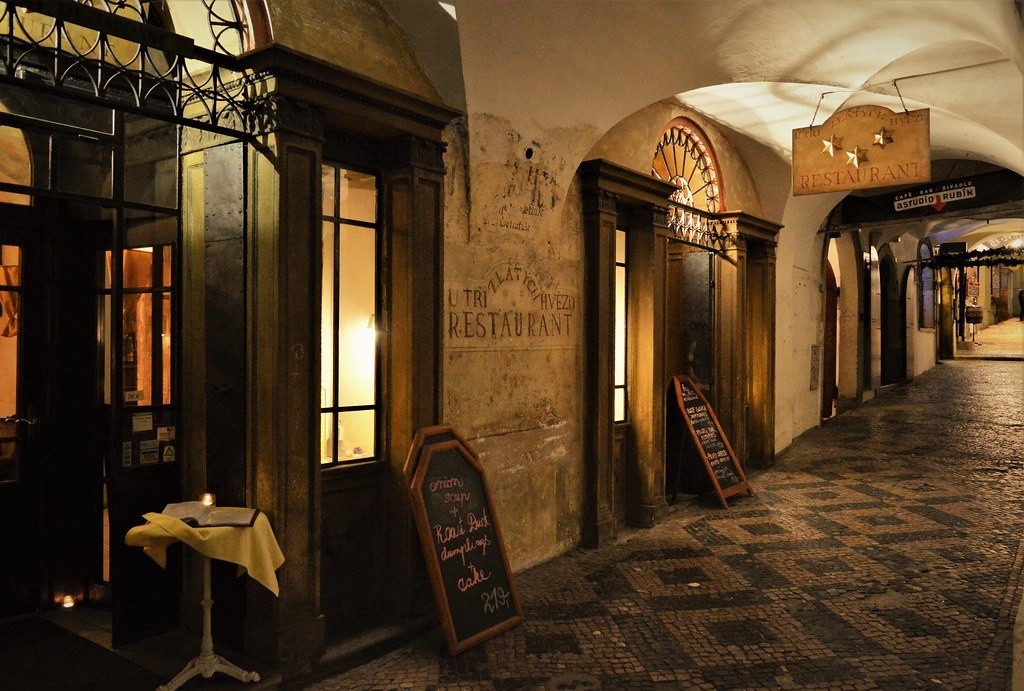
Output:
[409,439,525,656]
[672,374,755,509]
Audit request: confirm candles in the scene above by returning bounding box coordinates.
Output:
[202,493,216,511]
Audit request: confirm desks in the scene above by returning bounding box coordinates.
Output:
[137,507,273,691]
